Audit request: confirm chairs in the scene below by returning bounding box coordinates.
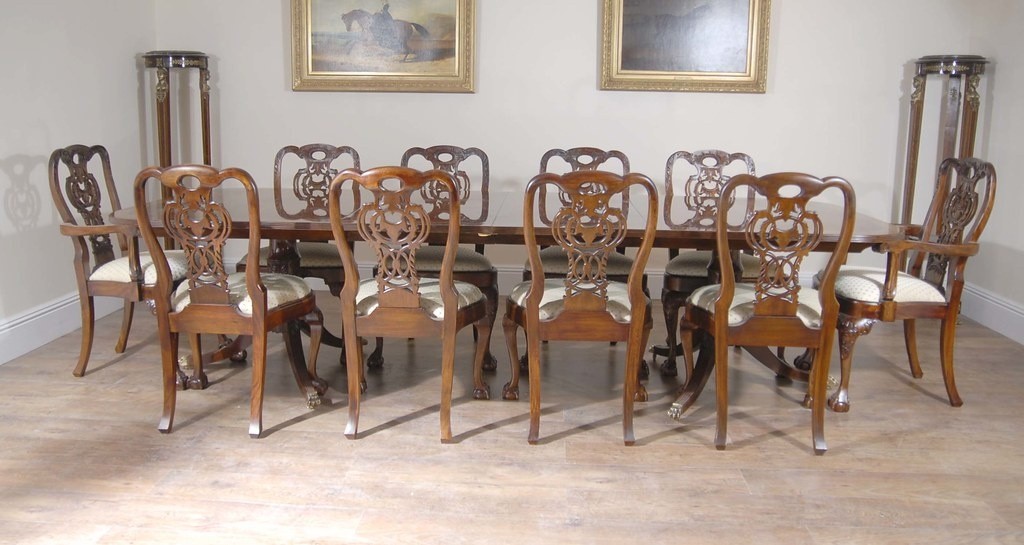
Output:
[133,144,856,456]
[48,145,248,392]
[794,156,997,413]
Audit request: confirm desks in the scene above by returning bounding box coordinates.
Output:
[106,188,905,420]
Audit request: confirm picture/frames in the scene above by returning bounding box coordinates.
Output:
[290,0,477,95]
[599,0,771,94]
